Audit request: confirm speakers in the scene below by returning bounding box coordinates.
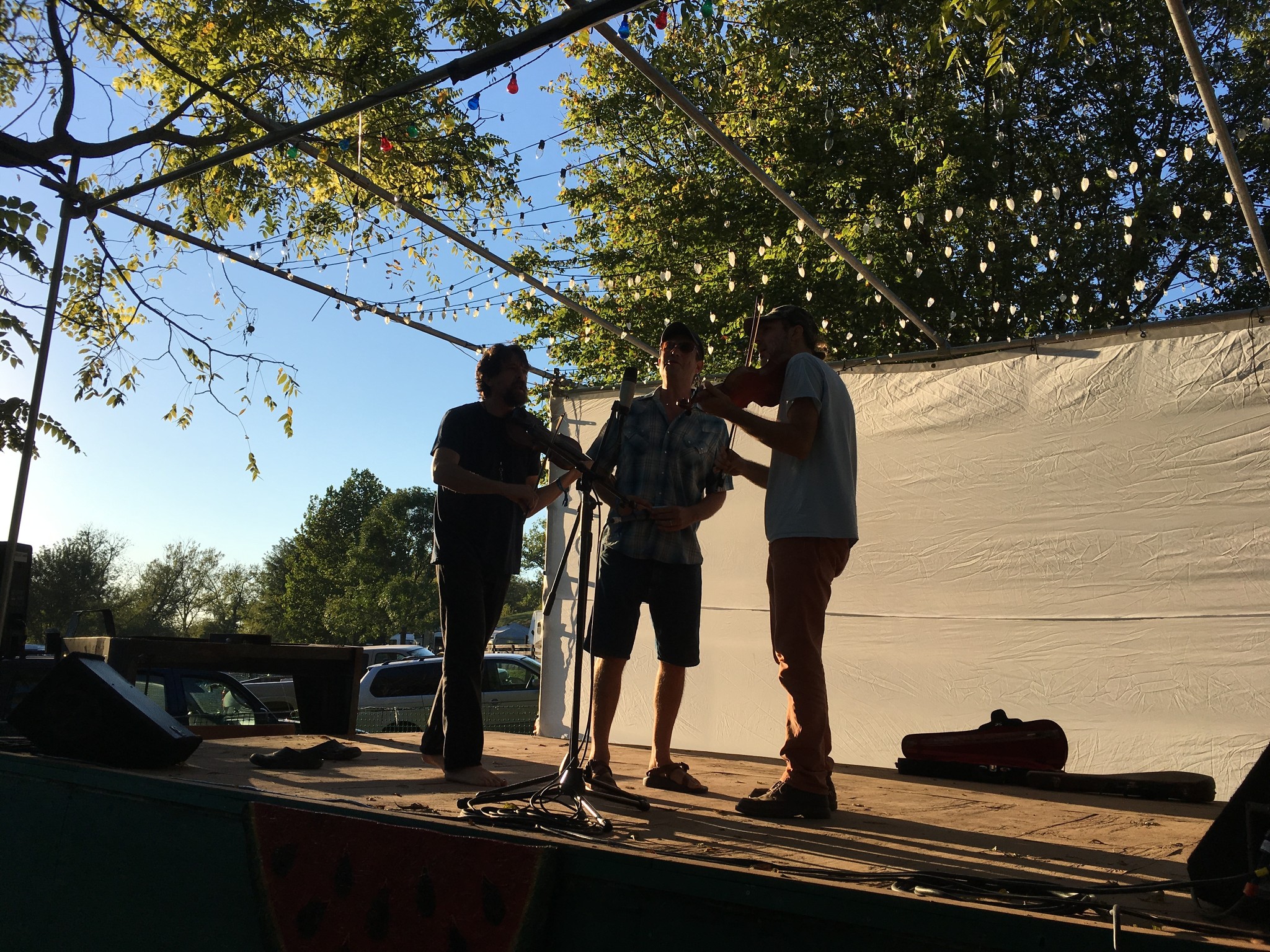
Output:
[1186,738,1270,918]
[23,652,203,769]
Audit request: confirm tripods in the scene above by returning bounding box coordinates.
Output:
[456,402,652,832]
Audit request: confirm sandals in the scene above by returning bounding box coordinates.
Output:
[585,760,622,796]
[643,761,708,794]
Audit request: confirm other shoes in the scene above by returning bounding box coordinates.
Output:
[748,770,838,812]
[735,780,830,820]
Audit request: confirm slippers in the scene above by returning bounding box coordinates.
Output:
[291,739,362,760]
[249,746,323,769]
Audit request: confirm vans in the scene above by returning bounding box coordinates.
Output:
[388,632,414,659]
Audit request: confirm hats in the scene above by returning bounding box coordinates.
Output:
[659,322,704,358]
[743,305,818,346]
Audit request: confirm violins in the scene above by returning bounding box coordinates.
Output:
[676,353,790,410]
[500,407,618,488]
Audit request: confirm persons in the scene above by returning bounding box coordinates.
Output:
[585,321,734,792]
[419,343,594,788]
[694,305,858,816]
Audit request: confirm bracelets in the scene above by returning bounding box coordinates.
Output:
[615,498,620,511]
[557,478,569,492]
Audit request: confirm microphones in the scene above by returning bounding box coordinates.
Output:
[618,367,637,414]
[1240,832,1270,902]
[610,508,650,523]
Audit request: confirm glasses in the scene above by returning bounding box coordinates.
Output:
[659,340,701,360]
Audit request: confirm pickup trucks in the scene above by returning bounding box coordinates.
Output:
[240,645,435,720]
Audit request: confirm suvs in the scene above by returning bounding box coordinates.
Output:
[355,656,541,733]
